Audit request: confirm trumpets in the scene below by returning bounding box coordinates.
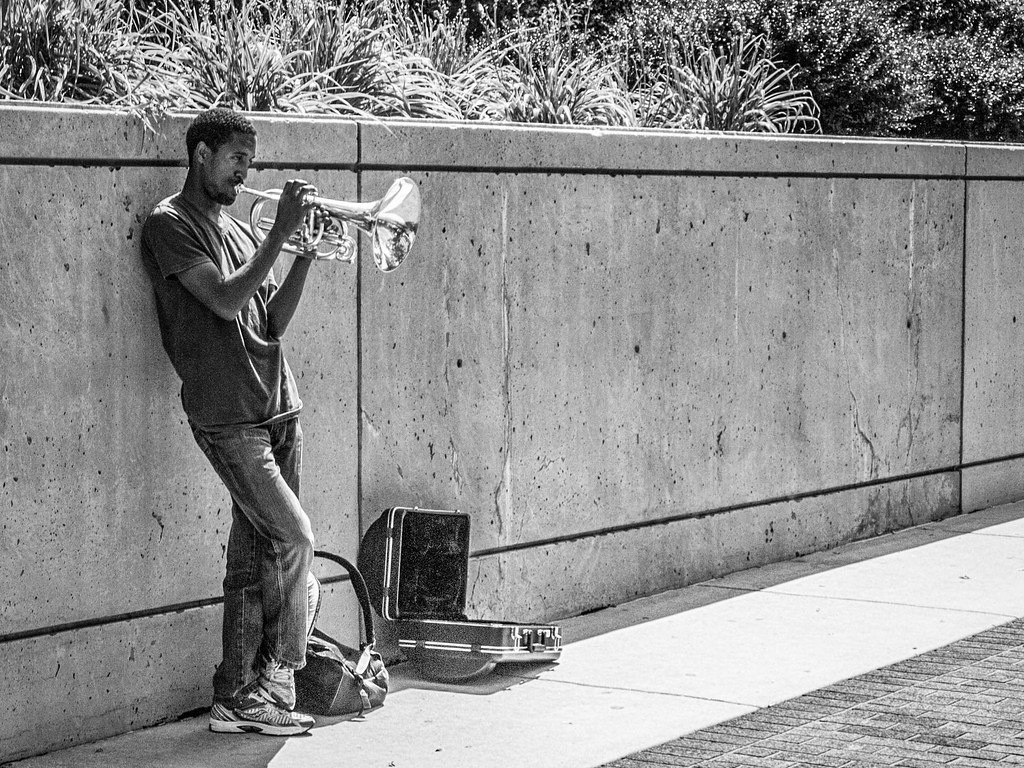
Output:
[231,176,425,274]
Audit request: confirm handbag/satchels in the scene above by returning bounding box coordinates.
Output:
[294,634,388,716]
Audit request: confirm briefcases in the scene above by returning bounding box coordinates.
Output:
[357,506,562,680]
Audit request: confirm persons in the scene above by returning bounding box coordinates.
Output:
[143,108,332,737]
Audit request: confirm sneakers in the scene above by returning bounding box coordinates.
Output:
[257,644,296,712]
[209,692,315,736]
